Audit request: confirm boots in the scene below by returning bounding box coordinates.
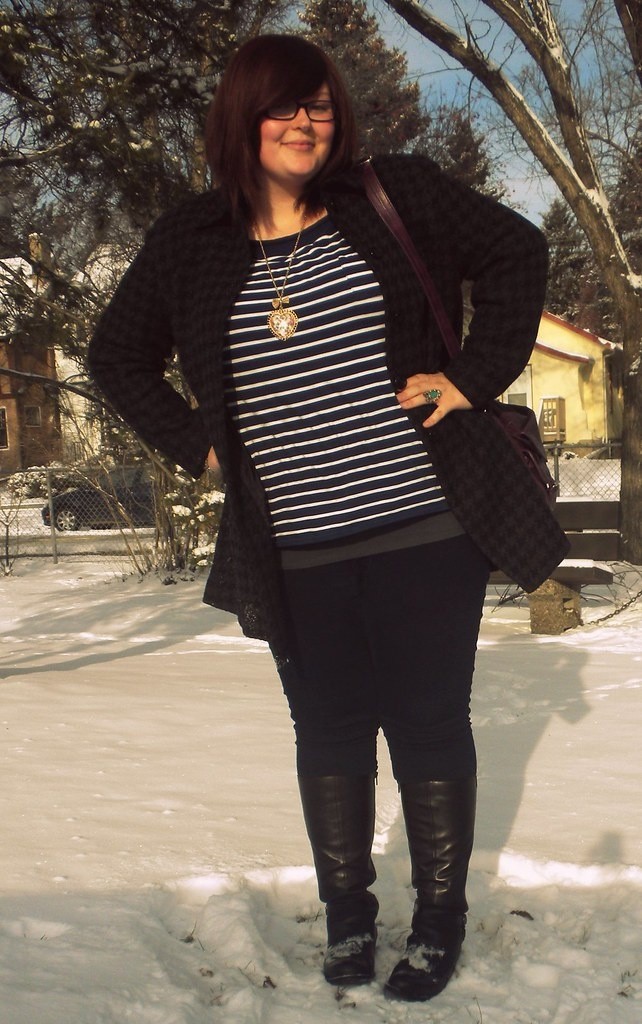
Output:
[387,775,482,1001]
[298,771,379,984]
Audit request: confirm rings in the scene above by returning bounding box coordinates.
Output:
[424,390,443,403]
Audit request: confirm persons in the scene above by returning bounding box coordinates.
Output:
[87,33,571,1002]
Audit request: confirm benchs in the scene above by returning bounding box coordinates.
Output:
[487,498,623,635]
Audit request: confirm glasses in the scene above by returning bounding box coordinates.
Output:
[268,98,338,123]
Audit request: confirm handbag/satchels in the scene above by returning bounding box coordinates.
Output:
[489,400,560,512]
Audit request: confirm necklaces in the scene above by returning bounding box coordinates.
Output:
[202,458,214,474]
[252,215,308,342]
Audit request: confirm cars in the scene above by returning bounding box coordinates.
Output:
[41,466,178,534]
